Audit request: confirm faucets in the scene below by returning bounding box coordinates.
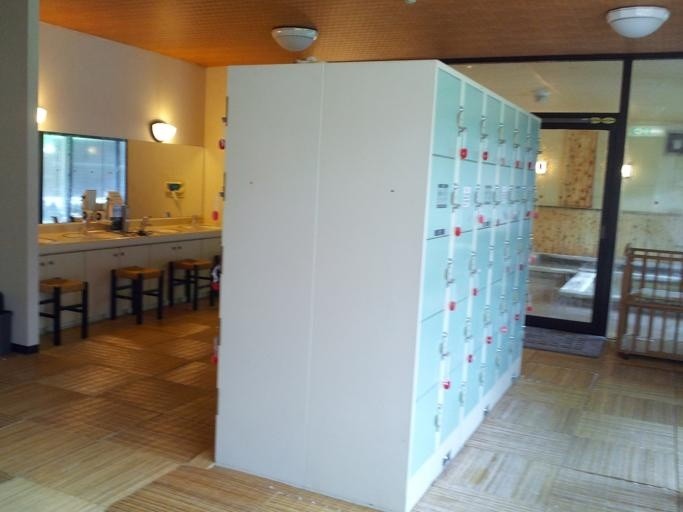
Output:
[139,215,152,230]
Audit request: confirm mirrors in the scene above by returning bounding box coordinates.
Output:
[40,131,128,224]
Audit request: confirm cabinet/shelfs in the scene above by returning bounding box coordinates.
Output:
[39,236,221,333]
[214,59,541,512]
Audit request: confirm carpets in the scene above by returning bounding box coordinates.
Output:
[523,325,606,358]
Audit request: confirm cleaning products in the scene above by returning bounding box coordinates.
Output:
[112,201,122,231]
[116,199,116,200]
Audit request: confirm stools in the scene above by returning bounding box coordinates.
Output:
[39,277,89,346]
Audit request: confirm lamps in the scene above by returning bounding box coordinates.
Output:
[605,6,672,39]
[535,160,548,175]
[271,27,320,51]
[37,106,47,123]
[152,122,177,142]
[621,164,632,177]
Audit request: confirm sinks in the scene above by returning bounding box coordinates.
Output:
[133,227,181,234]
